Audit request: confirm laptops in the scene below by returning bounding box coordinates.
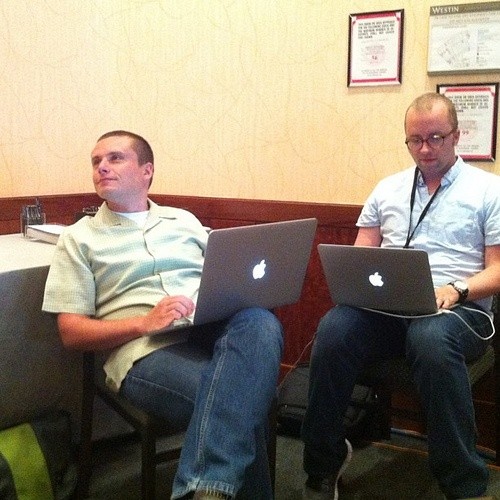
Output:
[147,218,318,337]
[317,244,461,316]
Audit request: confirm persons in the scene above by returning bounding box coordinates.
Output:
[42,131,285,498]
[302,92,500,500]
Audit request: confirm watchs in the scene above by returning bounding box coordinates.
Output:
[447,278,469,303]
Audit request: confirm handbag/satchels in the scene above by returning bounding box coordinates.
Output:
[277,362,386,450]
[0,417,79,500]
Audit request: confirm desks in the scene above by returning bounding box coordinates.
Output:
[0,232,136,446]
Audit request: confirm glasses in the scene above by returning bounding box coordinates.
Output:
[404,129,455,152]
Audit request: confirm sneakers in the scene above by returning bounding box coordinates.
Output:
[299,437,353,500]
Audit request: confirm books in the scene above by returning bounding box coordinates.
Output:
[25,225,69,245]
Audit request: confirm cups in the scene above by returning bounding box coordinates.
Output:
[20,213,46,238]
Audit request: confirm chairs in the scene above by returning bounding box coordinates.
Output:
[79,353,278,500]
[337,293,499,490]
